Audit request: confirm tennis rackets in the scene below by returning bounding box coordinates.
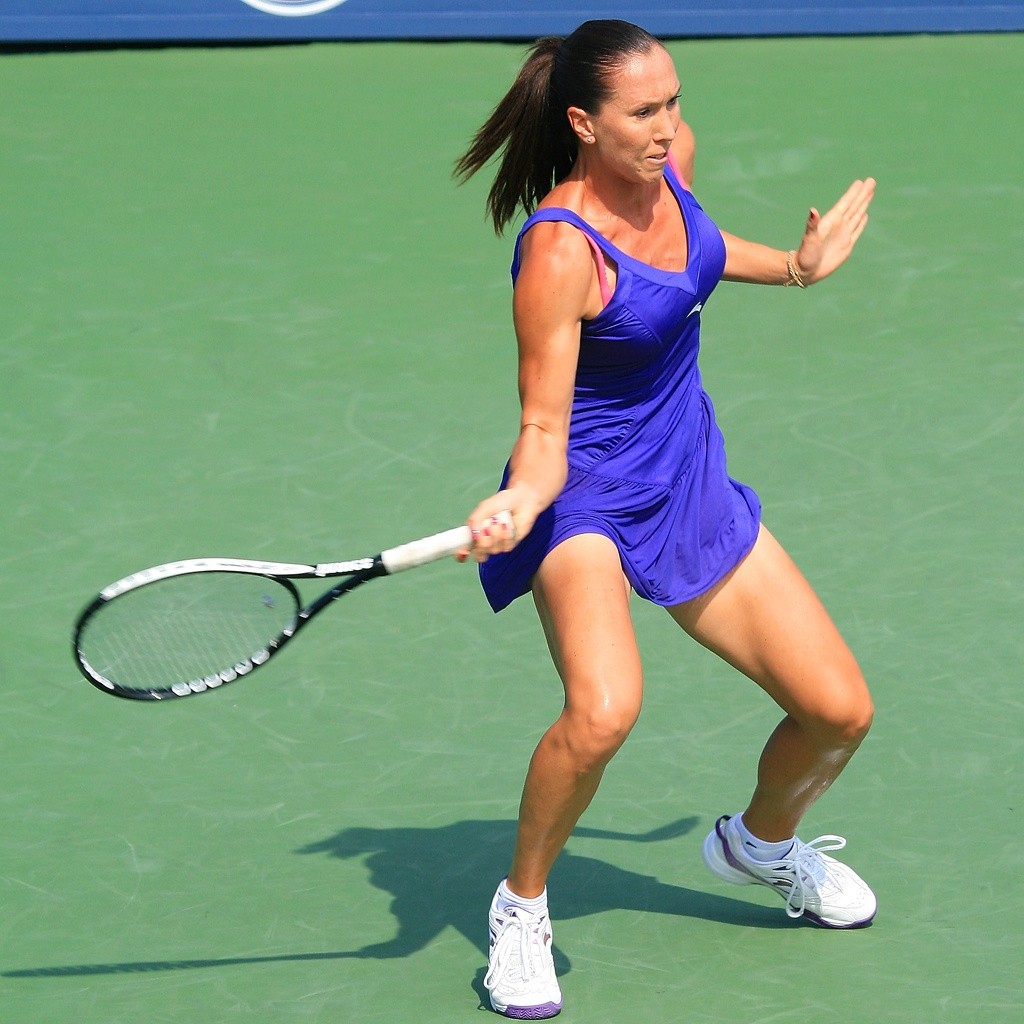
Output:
[70,508,511,701]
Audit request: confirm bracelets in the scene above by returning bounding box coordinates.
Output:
[783,246,805,293]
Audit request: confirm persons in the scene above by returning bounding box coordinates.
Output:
[457,19,877,1019]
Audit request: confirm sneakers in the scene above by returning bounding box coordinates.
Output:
[701,811,878,929]
[482,873,563,1021]
